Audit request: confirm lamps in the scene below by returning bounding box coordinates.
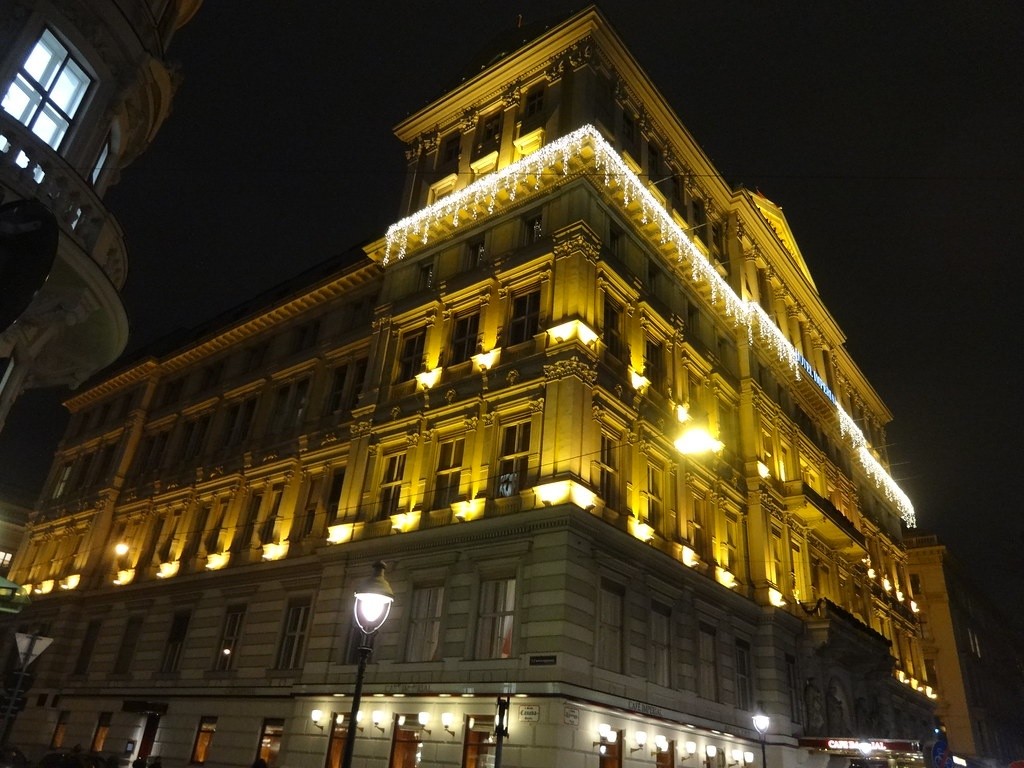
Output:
[356,711,366,733]
[372,711,385,732]
[13,362,489,597]
[631,731,649,752]
[635,384,936,698]
[728,750,743,767]
[555,333,564,343]
[419,712,432,734]
[586,337,597,349]
[593,724,617,754]
[336,714,349,729]
[311,710,324,729]
[741,752,754,768]
[586,496,598,513]
[605,738,607,739]
[441,713,455,735]
[682,741,696,761]
[651,735,668,756]
[703,745,717,764]
[542,496,555,508]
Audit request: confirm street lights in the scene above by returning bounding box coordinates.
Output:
[751,699,771,768]
[338,558,395,768]
